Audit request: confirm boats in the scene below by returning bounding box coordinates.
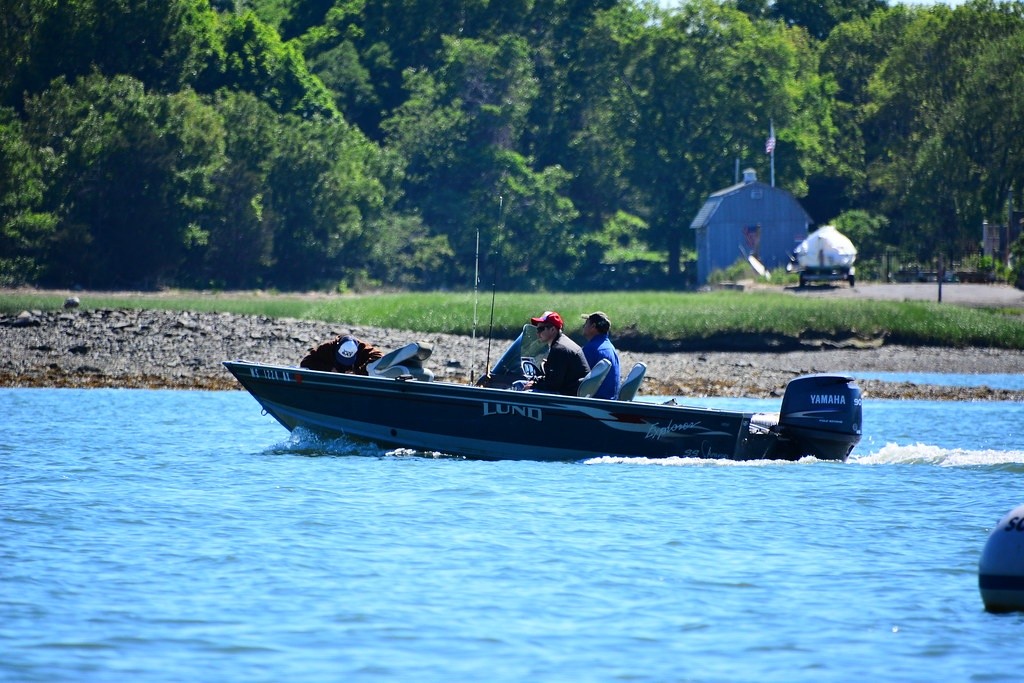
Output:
[797,228,858,272]
[222,324,864,464]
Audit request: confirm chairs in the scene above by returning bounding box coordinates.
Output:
[576,358,611,397]
[618,362,646,401]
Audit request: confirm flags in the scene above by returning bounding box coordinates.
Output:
[764,122,777,155]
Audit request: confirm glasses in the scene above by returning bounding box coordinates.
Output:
[536,326,549,333]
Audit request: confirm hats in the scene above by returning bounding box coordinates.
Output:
[581,311,611,329]
[531,311,563,329]
[336,335,360,363]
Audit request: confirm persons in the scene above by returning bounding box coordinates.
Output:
[521,310,591,397]
[580,310,621,400]
[300,333,384,376]
